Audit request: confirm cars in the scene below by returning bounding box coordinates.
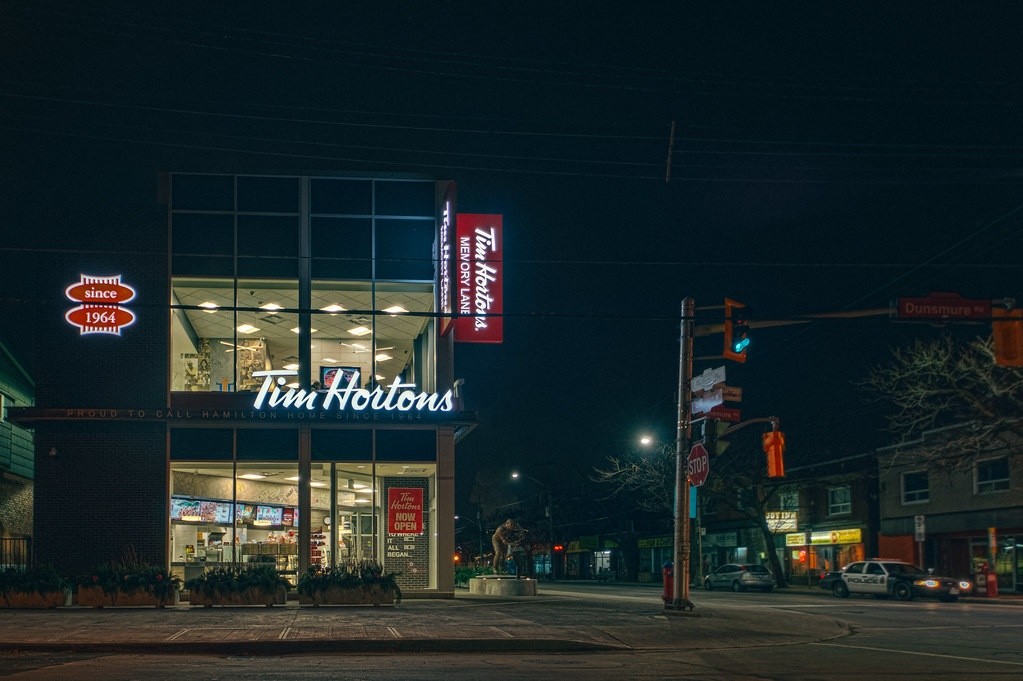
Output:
[704,563,777,595]
[817,557,971,602]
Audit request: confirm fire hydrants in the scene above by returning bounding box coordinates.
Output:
[661,558,674,607]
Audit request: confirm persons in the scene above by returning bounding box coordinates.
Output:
[491,518,521,574]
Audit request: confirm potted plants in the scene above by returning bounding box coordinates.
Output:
[0,553,73,609]
[184,562,293,609]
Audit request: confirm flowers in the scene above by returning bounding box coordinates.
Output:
[80,545,184,600]
[296,562,404,606]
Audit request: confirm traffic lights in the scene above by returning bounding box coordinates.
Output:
[723,297,751,365]
[701,417,730,457]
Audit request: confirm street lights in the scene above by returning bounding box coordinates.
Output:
[455,508,483,569]
[513,472,555,577]
[641,437,698,577]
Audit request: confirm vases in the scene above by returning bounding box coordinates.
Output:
[299,587,396,609]
[78,584,180,609]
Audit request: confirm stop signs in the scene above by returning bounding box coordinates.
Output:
[685,442,711,488]
